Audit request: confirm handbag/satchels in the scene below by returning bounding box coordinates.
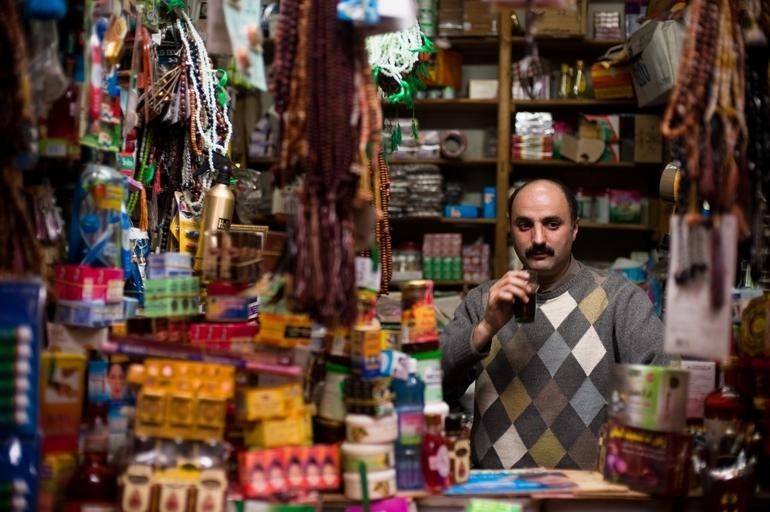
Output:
[624,17,686,110]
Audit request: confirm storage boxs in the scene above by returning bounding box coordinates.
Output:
[601,17,685,108]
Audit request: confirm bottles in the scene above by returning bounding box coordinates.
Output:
[390,356,472,493]
[558,60,588,98]
[402,278,440,353]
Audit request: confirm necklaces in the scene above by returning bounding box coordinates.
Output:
[163,11,232,214]
[657,1,756,310]
[356,18,430,113]
[269,0,397,325]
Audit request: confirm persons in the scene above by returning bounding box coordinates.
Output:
[437,178,681,473]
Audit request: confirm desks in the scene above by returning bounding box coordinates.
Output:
[320,463,672,511]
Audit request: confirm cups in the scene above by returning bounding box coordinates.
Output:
[512,269,537,323]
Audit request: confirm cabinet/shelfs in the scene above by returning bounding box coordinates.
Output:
[369,6,674,302]
